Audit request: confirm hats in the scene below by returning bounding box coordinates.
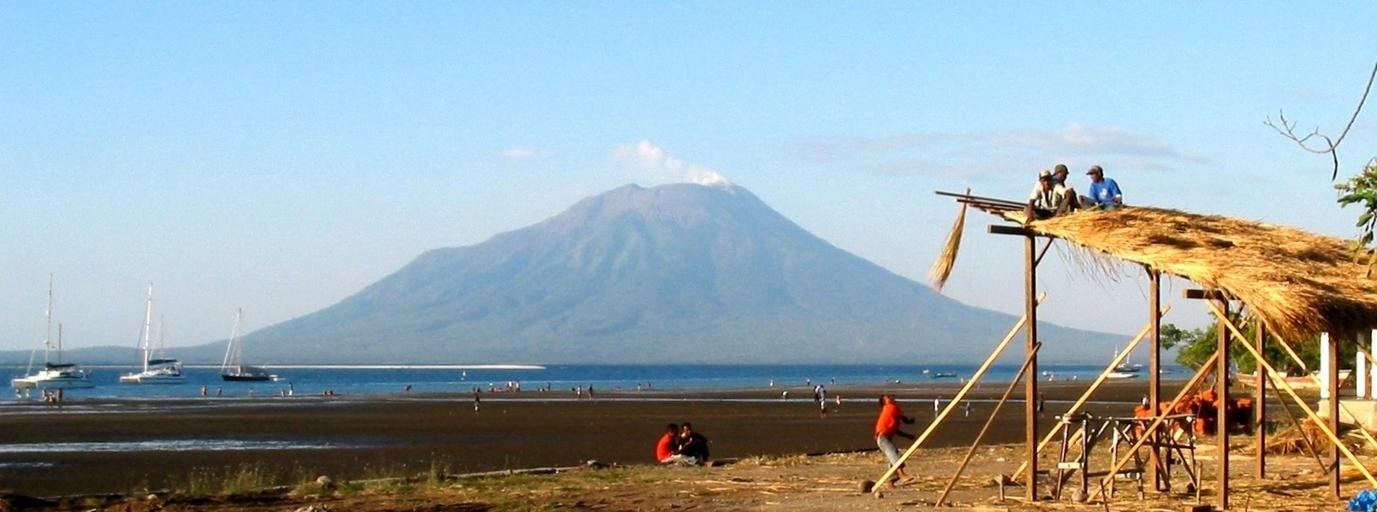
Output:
[1086,165,1102,175]
[1038,169,1051,180]
[1053,164,1069,176]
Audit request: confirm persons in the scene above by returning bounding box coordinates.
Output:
[770,373,841,413]
[1024,169,1074,225]
[679,423,709,463]
[281,389,286,399]
[14,385,65,406]
[288,382,294,398]
[934,399,939,420]
[216,386,223,397]
[1048,374,1080,383]
[965,402,971,417]
[637,382,651,391]
[201,384,208,397]
[1037,391,1045,417]
[587,383,593,401]
[405,384,411,395]
[873,394,918,486]
[1078,166,1122,212]
[655,423,697,465]
[577,385,581,398]
[1049,165,1081,210]
[470,376,551,412]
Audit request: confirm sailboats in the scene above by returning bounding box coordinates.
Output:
[1106,339,1145,373]
[215,302,290,387]
[118,281,188,388]
[10,271,101,395]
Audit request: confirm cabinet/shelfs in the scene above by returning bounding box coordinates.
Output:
[1055,414,1202,501]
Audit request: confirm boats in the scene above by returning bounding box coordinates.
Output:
[1097,372,1141,382]
[1236,364,1355,396]
[924,368,962,381]
[1039,369,1060,378]
[1158,368,1174,377]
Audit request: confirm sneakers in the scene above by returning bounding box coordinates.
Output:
[893,474,915,486]
